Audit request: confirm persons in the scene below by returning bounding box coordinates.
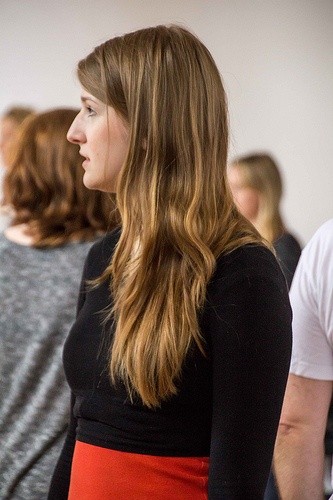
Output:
[0,108,333,500]
[47,21,293,500]
